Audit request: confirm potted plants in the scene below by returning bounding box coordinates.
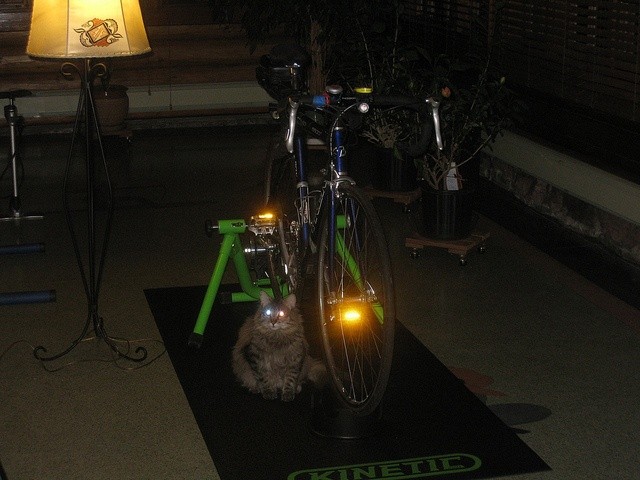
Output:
[93,58,131,129]
[323,0,415,190]
[399,0,467,241]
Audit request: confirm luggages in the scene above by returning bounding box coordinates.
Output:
[257,48,377,101]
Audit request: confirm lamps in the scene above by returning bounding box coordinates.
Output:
[18,0,154,369]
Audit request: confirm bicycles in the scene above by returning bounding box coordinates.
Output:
[248,42,443,418]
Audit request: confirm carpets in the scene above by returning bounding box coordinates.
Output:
[142,282,553,476]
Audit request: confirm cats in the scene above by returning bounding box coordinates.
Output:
[227,291,329,402]
